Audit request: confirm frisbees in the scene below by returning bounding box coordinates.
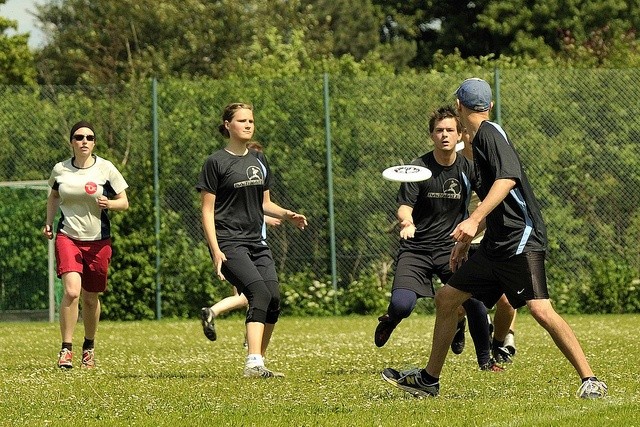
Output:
[383,165,432,183]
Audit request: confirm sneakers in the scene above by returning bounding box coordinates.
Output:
[376,313,393,348]
[491,345,512,365]
[57,347,73,368]
[243,365,283,379]
[451,319,466,355]
[504,342,517,356]
[199,307,217,341]
[81,349,95,370]
[487,320,495,349]
[481,359,506,371]
[381,367,440,397]
[577,380,606,400]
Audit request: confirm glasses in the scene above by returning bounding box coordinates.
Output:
[73,134,95,141]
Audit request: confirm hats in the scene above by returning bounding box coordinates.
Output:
[70,121,96,141]
[453,78,493,114]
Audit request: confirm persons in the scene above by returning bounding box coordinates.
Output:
[451,114,515,364]
[43,122,129,366]
[375,104,480,351]
[196,103,307,378]
[200,142,282,349]
[381,78,607,402]
